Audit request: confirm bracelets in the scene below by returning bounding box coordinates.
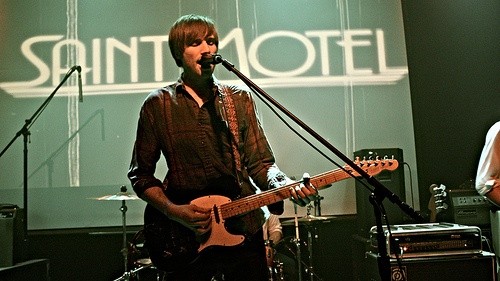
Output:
[164,201,172,216]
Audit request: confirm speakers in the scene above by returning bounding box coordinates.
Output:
[0,203,25,267]
[353,148,406,230]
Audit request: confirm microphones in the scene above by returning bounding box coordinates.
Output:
[201,53,222,64]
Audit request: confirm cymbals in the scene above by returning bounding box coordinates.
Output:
[280,215,337,226]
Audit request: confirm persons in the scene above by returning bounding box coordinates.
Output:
[127,14,318,281]
[475,120,500,209]
[262,212,283,267]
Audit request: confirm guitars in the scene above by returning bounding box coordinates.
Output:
[143,154,399,273]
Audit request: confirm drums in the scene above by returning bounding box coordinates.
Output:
[135,259,159,281]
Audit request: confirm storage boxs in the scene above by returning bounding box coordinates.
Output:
[365,251,498,281]
